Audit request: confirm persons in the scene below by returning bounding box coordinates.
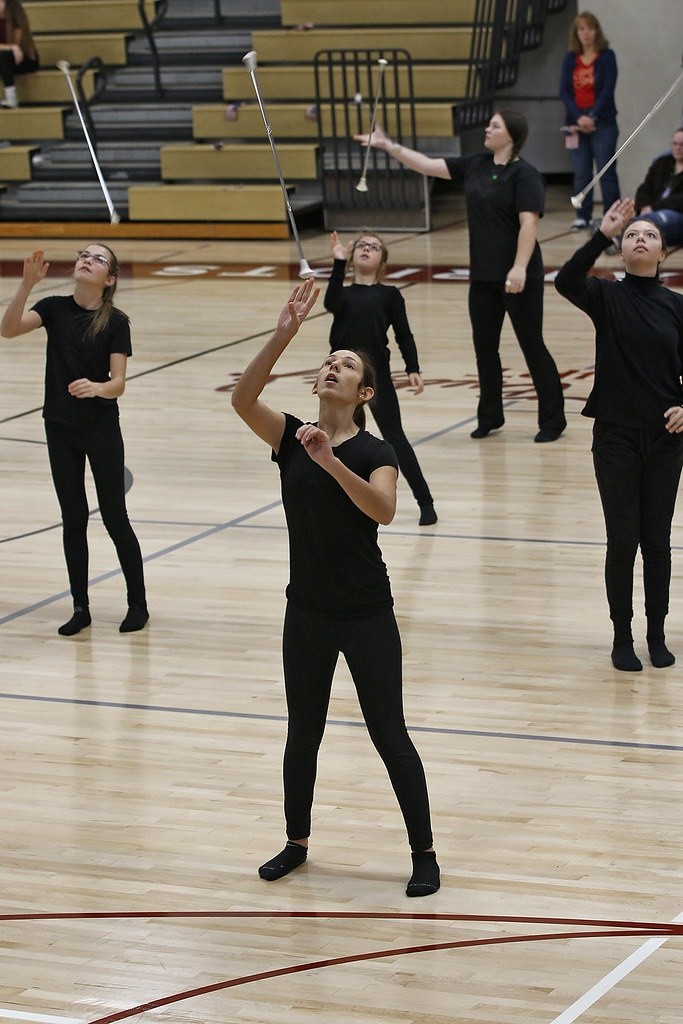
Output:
[555,194,683,672]
[354,110,568,443]
[1,243,150,637]
[559,11,621,231]
[591,128,683,255]
[232,277,441,898]
[323,230,437,527]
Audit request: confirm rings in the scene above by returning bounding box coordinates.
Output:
[505,281,511,287]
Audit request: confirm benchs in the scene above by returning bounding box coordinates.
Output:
[192,101,463,139]
[0,145,42,183]
[158,144,325,181]
[128,183,296,222]
[0,69,107,105]
[32,29,136,68]
[0,106,73,142]
[282,0,533,26]
[222,65,482,99]
[22,0,156,32]
[251,26,508,62]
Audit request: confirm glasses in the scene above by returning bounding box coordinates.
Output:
[353,241,383,253]
[77,251,110,269]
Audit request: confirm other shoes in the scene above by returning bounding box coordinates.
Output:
[571,219,587,233]
